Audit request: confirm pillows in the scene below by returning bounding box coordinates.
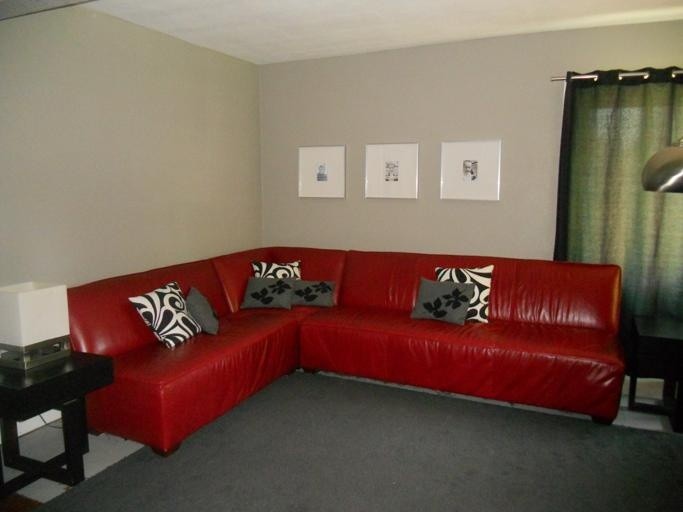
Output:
[127,281,200,350]
[184,287,219,334]
[242,259,334,309]
[433,265,494,323]
[411,277,475,325]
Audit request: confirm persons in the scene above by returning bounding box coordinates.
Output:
[316,165,327,181]
[463,160,472,184]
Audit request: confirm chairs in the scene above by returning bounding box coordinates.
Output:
[621,302,683,433]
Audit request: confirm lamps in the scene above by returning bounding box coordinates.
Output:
[0,282,71,371]
[641,137,683,193]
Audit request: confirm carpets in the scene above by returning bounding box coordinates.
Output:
[32,370,682,512]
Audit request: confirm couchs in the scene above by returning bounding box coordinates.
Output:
[68,247,626,457]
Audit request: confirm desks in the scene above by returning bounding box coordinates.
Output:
[1,351,114,512]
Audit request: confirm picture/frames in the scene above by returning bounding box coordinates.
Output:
[298,146,346,199]
[440,141,502,201]
[364,144,419,199]
